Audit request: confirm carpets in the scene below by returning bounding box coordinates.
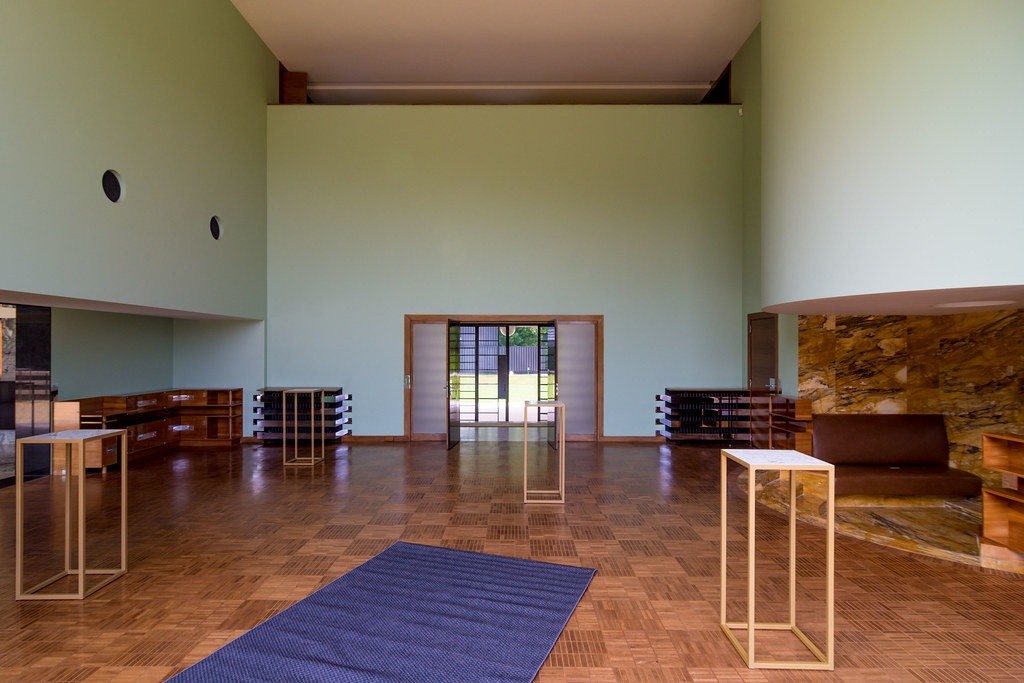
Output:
[164,540,598,683]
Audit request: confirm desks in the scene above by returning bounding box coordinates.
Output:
[282,388,326,466]
[524,401,566,504]
[15,429,128,600]
[720,449,835,672]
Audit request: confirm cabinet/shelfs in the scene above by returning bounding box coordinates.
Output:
[769,395,812,451]
[53,388,243,475]
[981,431,1024,555]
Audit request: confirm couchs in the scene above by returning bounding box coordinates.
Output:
[810,413,983,498]
